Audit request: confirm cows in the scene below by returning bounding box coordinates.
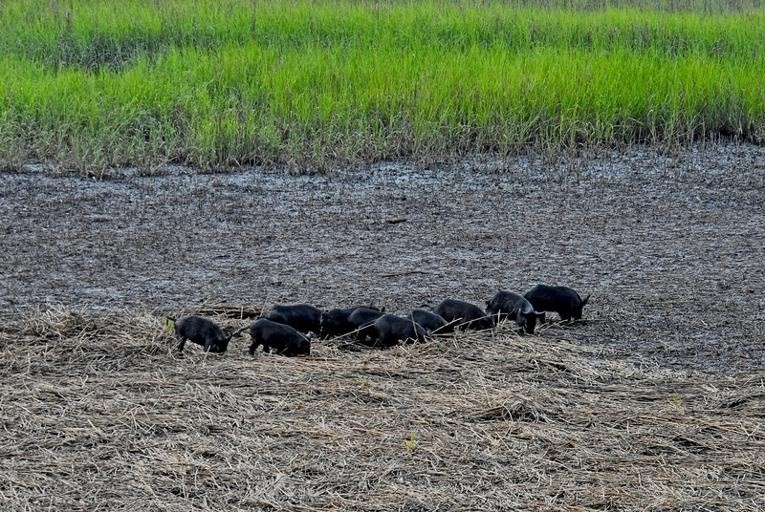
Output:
[166,316,233,359]
[319,307,386,341]
[374,314,435,350]
[485,294,499,327]
[434,298,496,334]
[408,309,456,340]
[346,308,386,346]
[269,304,328,337]
[492,290,547,334]
[524,284,592,325]
[233,319,312,357]
